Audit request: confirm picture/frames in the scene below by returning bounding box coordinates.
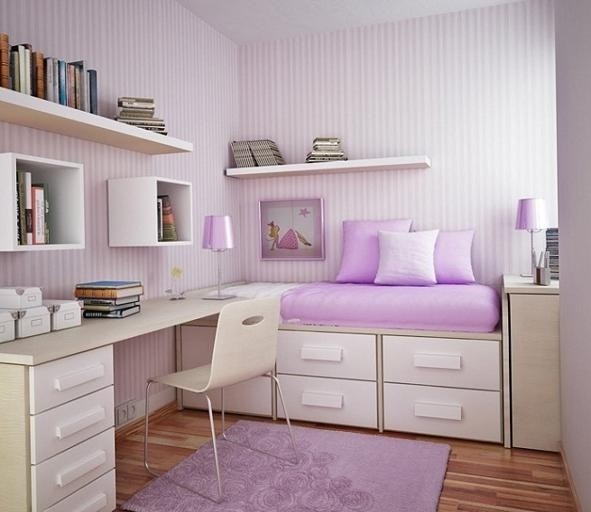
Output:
[258,197,326,262]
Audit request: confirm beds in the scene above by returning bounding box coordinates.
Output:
[174,281,504,444]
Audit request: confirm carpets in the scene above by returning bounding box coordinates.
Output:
[119,419,452,512]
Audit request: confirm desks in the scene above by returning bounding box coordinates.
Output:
[1,295,255,512]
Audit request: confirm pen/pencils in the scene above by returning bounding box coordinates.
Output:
[531,251,550,269]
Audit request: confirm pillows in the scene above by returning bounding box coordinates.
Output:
[335,218,477,287]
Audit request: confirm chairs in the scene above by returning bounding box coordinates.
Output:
[144,295,300,503]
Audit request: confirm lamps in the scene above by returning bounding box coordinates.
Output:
[202,215,238,300]
[515,197,547,278]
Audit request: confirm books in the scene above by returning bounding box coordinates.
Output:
[230,139,286,167]
[74,280,145,319]
[305,136,350,163]
[546,226,561,280]
[114,96,169,136]
[158,194,180,243]
[16,169,52,246]
[0,32,99,116]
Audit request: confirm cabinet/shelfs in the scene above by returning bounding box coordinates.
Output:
[108,176,195,248]
[502,275,564,454]
[1,152,86,253]
